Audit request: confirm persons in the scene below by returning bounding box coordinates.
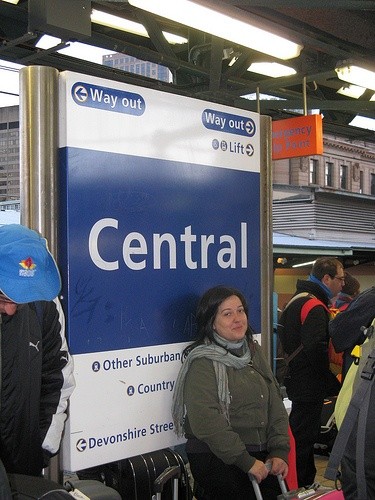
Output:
[0,222,76,500]
[171,284,292,500]
[327,274,374,500]
[277,256,346,492]
